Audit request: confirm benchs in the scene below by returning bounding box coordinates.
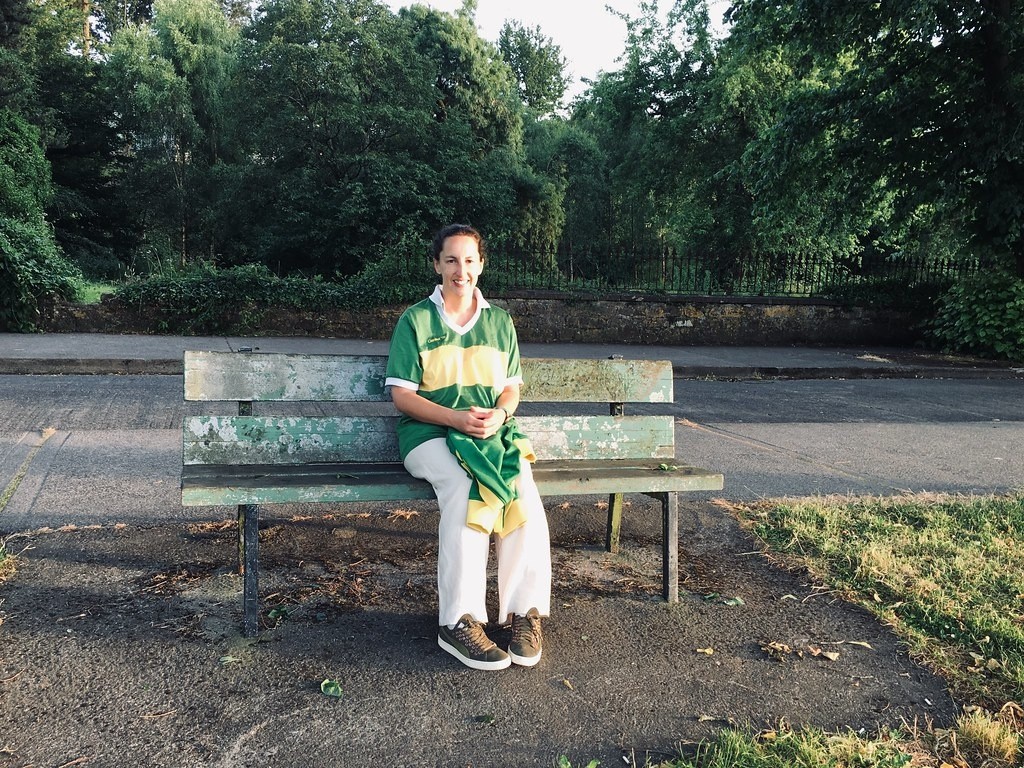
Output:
[180,346,723,639]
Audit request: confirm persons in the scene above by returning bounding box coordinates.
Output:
[384,223,552,670]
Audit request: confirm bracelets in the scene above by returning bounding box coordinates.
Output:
[493,406,511,425]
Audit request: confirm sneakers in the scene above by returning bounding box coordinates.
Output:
[508,607,542,666]
[437,612,511,671]
